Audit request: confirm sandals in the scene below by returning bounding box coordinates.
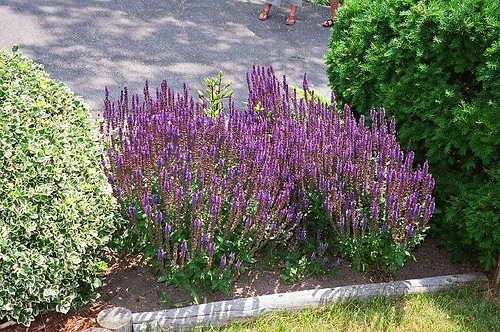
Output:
[322,19,334,27]
[258,11,269,21]
[286,15,296,26]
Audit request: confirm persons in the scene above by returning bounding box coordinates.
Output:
[257,0,303,26]
[321,0,348,28]
